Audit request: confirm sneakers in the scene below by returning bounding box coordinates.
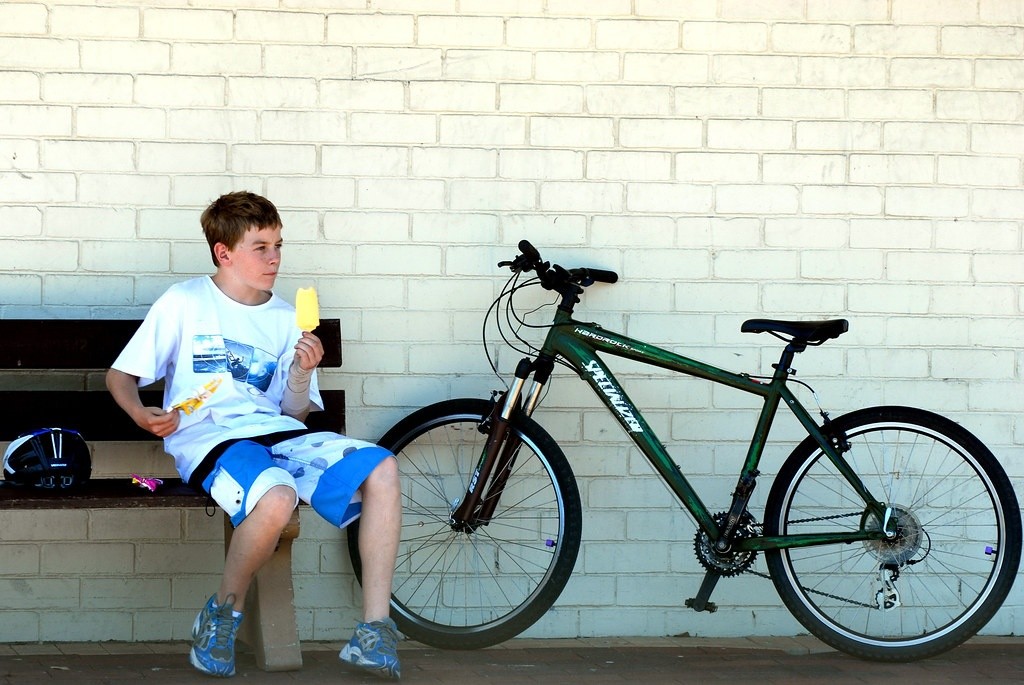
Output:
[189,591,244,677]
[338,616,404,680]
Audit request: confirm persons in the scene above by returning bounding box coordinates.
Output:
[105,190,404,681]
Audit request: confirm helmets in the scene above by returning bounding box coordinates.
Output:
[1,428,92,500]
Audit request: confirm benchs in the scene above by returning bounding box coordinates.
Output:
[0,318,346,669]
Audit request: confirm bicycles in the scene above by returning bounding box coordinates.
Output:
[348,238,1022,664]
